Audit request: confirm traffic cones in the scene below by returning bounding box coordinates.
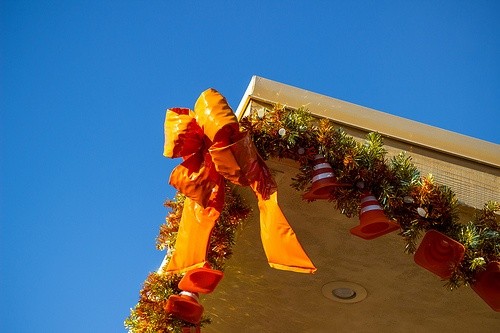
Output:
[410,228,466,282]
[175,261,223,297]
[300,151,351,205]
[343,181,402,245]
[160,288,203,328]
